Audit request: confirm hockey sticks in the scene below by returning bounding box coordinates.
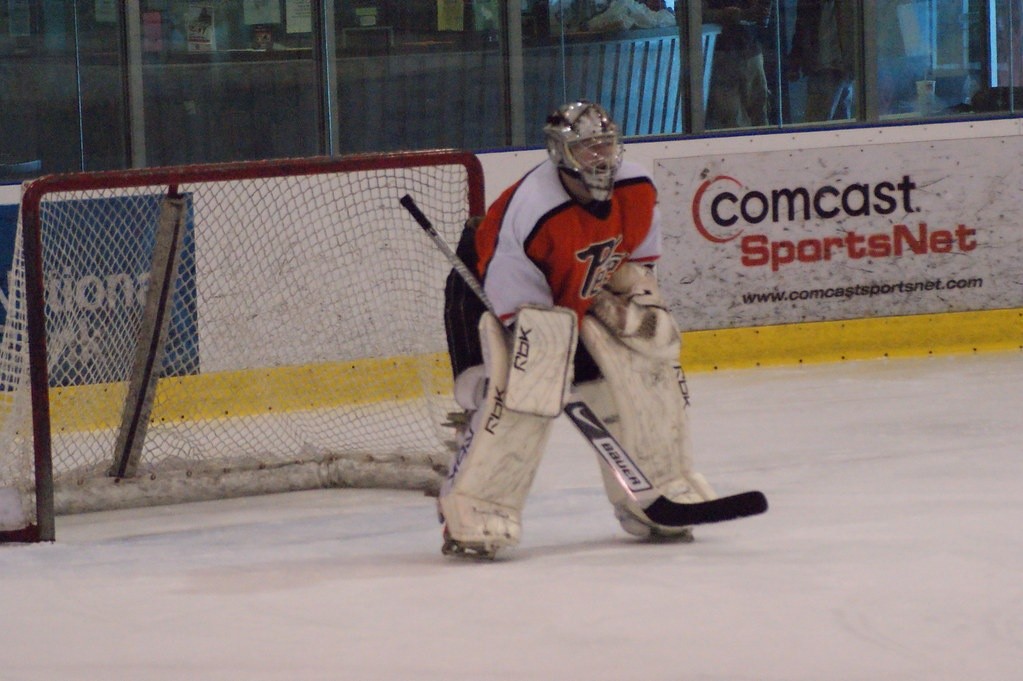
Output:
[398,193,769,528]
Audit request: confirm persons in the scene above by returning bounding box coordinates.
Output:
[437,102,717,546]
[674,0,771,130]
[781,0,857,123]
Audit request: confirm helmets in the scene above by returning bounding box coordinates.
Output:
[542,98,623,202]
